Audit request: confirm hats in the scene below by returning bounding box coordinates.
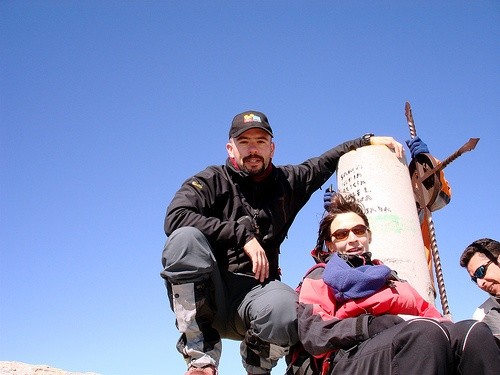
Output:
[228,110,274,139]
[322,252,392,304]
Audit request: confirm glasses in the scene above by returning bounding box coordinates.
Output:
[330,224,367,240]
[471,260,493,283]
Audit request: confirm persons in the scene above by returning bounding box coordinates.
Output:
[460,238,500,340]
[298,193,500,375]
[159,110,405,375]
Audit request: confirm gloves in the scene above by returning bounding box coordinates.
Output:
[323,187,337,214]
[406,136,431,160]
[367,313,405,337]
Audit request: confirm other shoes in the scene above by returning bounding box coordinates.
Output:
[182,362,221,375]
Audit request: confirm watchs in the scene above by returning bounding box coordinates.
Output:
[364,133,374,143]
